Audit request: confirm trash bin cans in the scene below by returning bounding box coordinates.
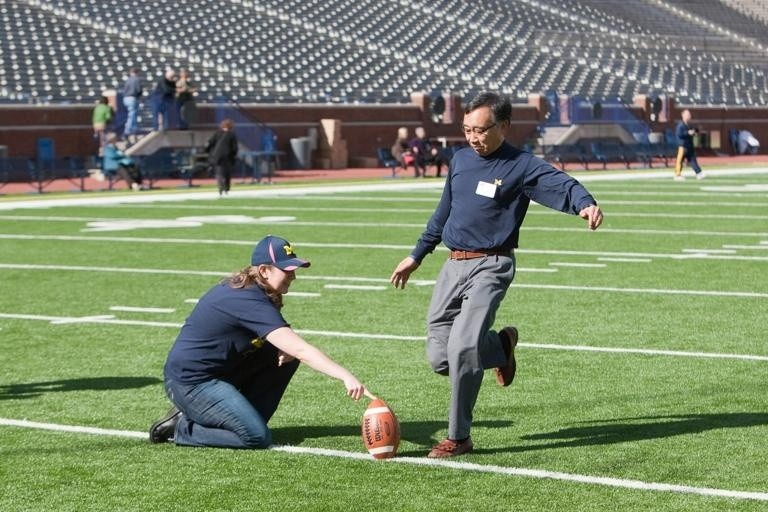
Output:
[290,137,315,169]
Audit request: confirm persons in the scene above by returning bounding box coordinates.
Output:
[392,127,443,177]
[209,119,238,192]
[433,97,444,123]
[148,234,377,450]
[123,69,144,142]
[673,109,707,182]
[388,91,605,461]
[93,97,114,156]
[647,91,663,121]
[104,85,124,141]
[155,68,192,129]
[104,133,143,190]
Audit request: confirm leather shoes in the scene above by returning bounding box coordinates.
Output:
[149,405,186,443]
[428,435,472,457]
[496,327,519,387]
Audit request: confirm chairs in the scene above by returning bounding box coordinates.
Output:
[0,1,767,196]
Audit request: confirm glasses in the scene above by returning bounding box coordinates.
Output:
[461,123,497,135]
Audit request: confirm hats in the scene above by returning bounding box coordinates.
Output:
[251,235,311,271]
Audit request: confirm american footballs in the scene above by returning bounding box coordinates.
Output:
[362,399,401,459]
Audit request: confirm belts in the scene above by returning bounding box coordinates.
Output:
[449,249,487,259]
